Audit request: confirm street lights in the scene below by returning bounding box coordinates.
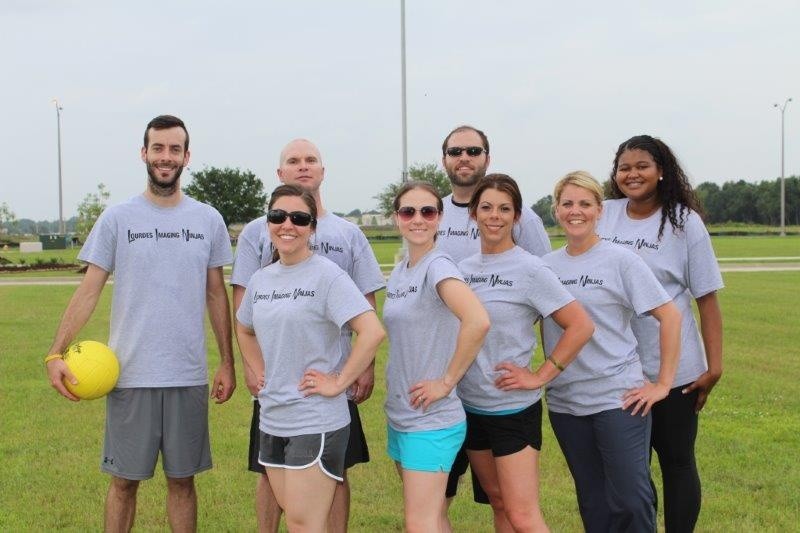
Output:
[773,97,792,238]
[51,96,65,235]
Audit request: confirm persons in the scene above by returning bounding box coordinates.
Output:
[49,116,237,533]
[228,125,725,533]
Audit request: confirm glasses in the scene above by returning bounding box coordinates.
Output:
[397,206,440,221]
[445,146,487,157]
[267,209,314,227]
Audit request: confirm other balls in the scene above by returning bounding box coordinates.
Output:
[61,339,122,398]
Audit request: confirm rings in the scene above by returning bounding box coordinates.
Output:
[312,381,316,387]
[418,396,423,401]
[641,399,645,403]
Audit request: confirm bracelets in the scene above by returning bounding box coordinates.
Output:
[45,354,63,368]
[548,356,564,372]
